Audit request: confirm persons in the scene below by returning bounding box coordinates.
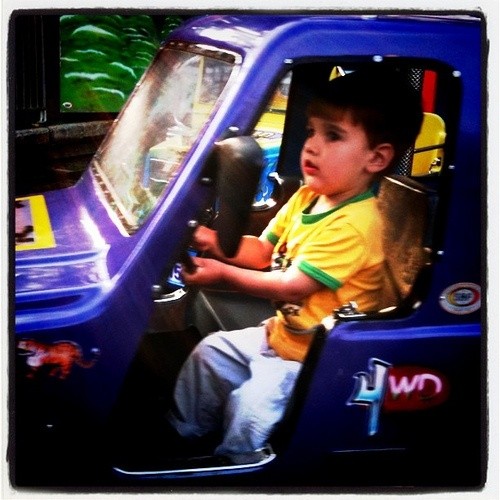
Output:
[109,62,424,476]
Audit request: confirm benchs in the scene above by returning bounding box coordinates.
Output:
[184,169,439,387]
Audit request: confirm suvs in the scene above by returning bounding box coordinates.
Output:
[12,13,488,485]
